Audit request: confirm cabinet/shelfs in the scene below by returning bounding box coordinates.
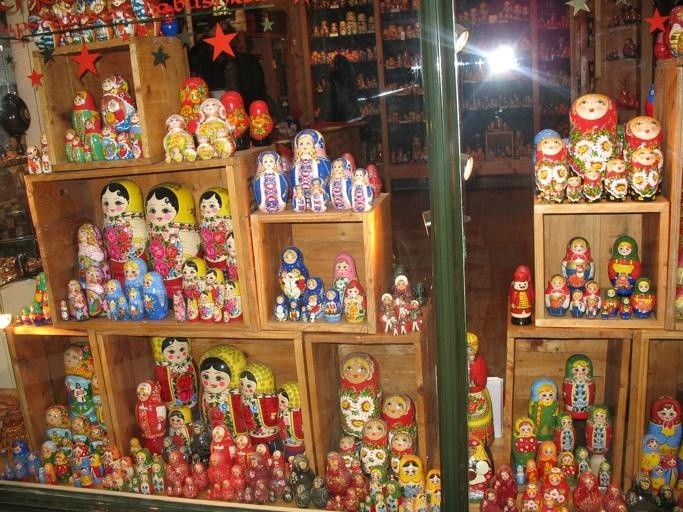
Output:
[0,0,681,510]
